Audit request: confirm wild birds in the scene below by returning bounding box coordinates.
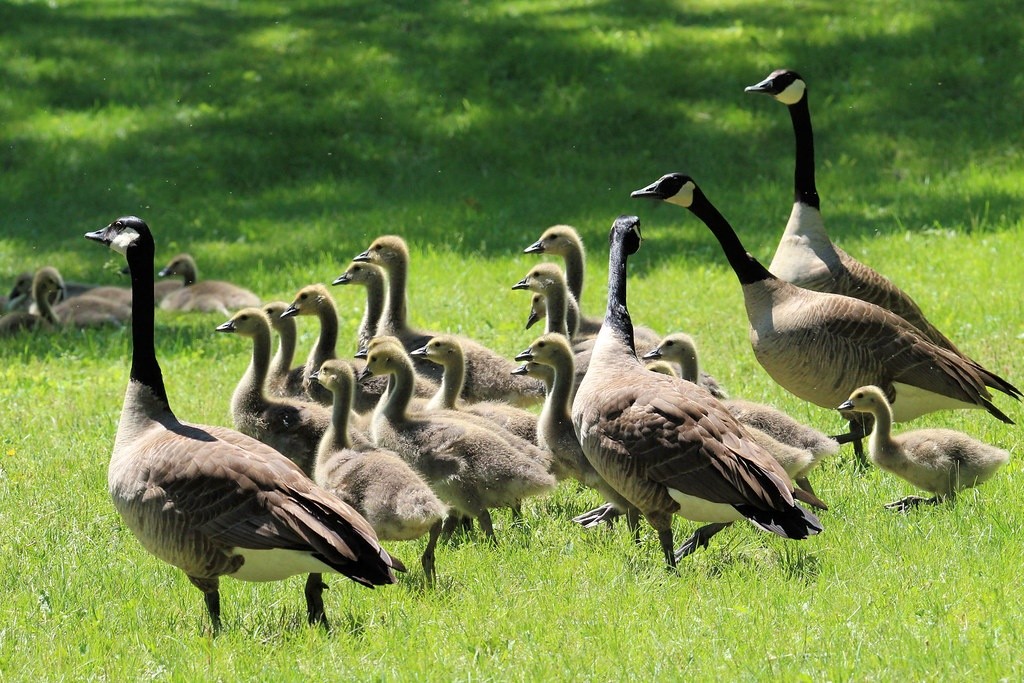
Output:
[837,384,1010,517]
[746,69,984,370]
[0,254,259,338]
[510,224,727,556]
[641,334,840,519]
[85,216,408,644]
[280,285,441,419]
[354,335,556,547]
[630,172,1024,474]
[332,235,547,408]
[216,302,371,488]
[308,359,447,589]
